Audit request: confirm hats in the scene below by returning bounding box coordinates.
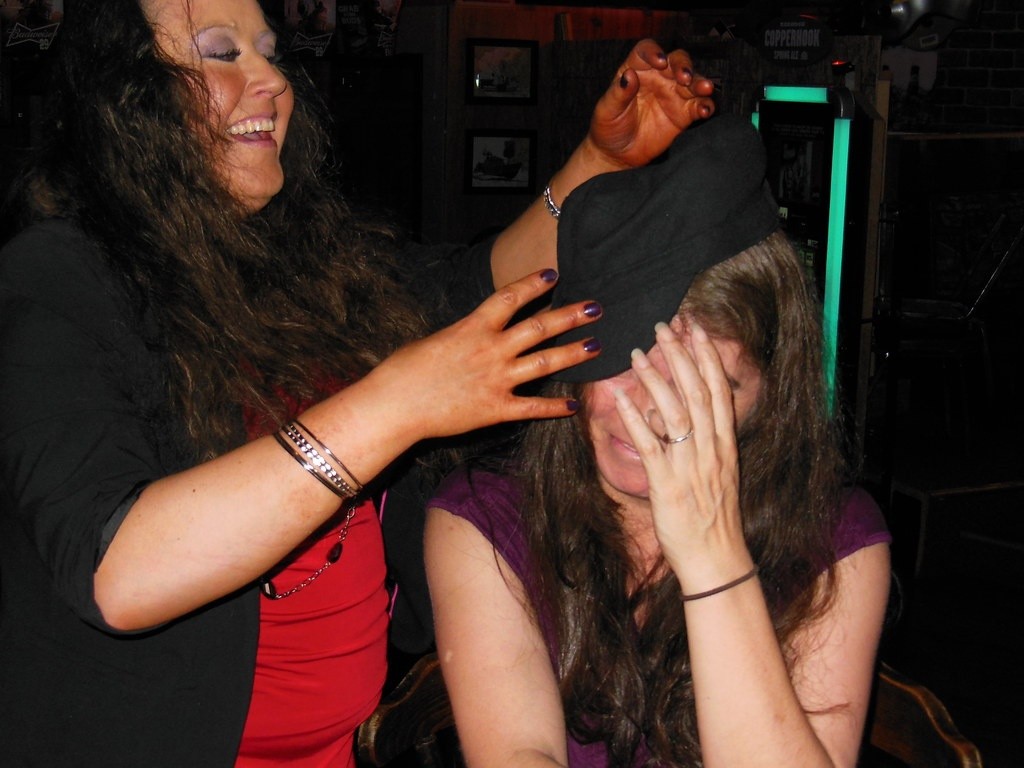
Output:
[552,112,780,381]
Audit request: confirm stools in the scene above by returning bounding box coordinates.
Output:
[888,464,1023,580]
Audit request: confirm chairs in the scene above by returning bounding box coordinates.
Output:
[899,213,1023,467]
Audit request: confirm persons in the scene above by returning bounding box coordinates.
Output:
[0,0,716,768]
[422,108,889,768]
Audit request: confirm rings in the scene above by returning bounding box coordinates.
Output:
[669,430,692,443]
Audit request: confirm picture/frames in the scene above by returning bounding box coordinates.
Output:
[465,127,536,194]
[462,37,542,105]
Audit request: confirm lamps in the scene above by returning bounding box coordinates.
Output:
[863,0,976,51]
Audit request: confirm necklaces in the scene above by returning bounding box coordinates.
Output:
[258,493,357,599]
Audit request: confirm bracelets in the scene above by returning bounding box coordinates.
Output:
[678,564,760,601]
[273,419,363,501]
[543,183,562,220]
